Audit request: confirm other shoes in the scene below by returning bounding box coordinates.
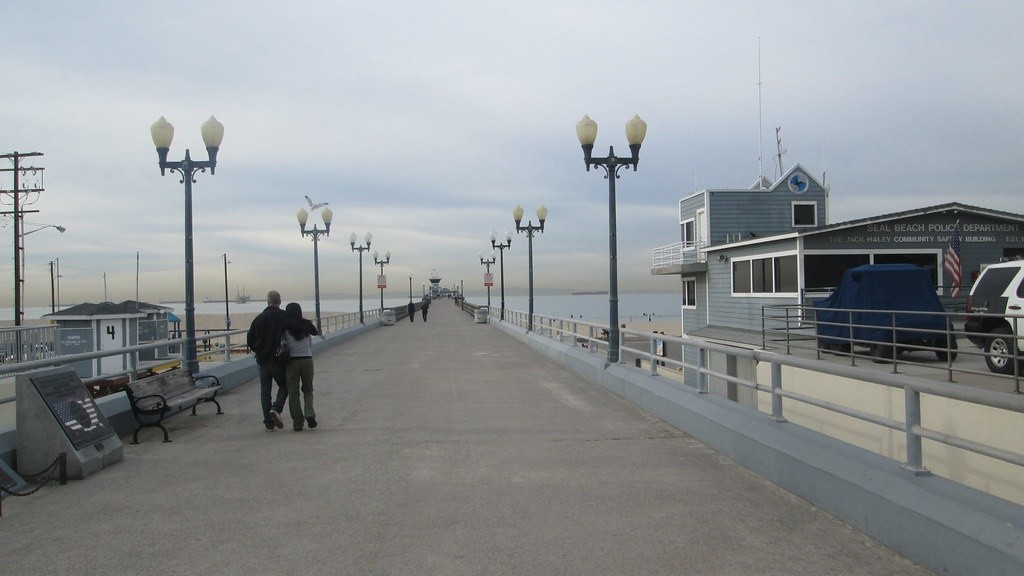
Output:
[306,416,317,428]
[270,409,284,429]
[267,426,274,431]
[295,427,301,431]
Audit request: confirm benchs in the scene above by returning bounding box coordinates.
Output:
[123,364,224,443]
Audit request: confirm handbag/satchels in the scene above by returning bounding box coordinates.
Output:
[274,331,290,362]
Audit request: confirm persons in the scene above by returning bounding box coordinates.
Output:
[407,300,415,322]
[244,290,318,432]
[454,296,458,305]
[445,293,451,298]
[421,298,429,322]
[283,303,316,430]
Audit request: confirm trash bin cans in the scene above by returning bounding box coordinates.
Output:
[383,309,395,325]
[474,309,488,324]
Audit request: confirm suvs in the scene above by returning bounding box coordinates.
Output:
[963,260,1024,374]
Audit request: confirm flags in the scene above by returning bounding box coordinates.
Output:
[943,224,962,300]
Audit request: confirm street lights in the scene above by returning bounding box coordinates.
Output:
[296,205,333,335]
[373,250,391,312]
[478,250,496,315]
[149,114,225,374]
[347,230,373,325]
[17,224,66,327]
[489,228,512,321]
[512,202,548,331]
[407,273,414,303]
[575,112,647,366]
